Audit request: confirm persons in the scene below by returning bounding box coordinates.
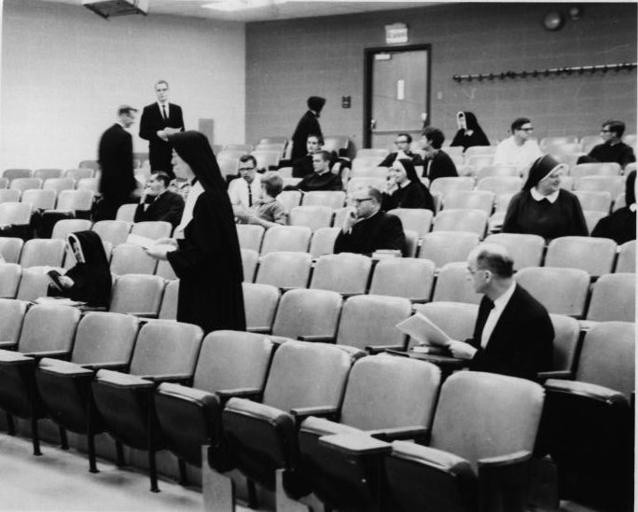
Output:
[501,153,588,245]
[591,170,636,243]
[134,170,184,228]
[450,112,491,154]
[332,185,408,257]
[99,106,138,220]
[290,96,351,191]
[142,130,245,331]
[47,231,111,311]
[495,119,542,163]
[443,242,556,385]
[139,80,183,179]
[228,154,289,227]
[369,125,458,217]
[578,120,635,170]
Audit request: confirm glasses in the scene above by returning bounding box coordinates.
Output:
[600,129,613,133]
[355,199,372,203]
[517,128,534,132]
[306,141,320,145]
[155,88,168,92]
[394,141,408,144]
[239,166,256,171]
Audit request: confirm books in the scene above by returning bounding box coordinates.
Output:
[410,345,444,355]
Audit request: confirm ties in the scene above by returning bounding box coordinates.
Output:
[248,185,253,207]
[162,105,168,125]
